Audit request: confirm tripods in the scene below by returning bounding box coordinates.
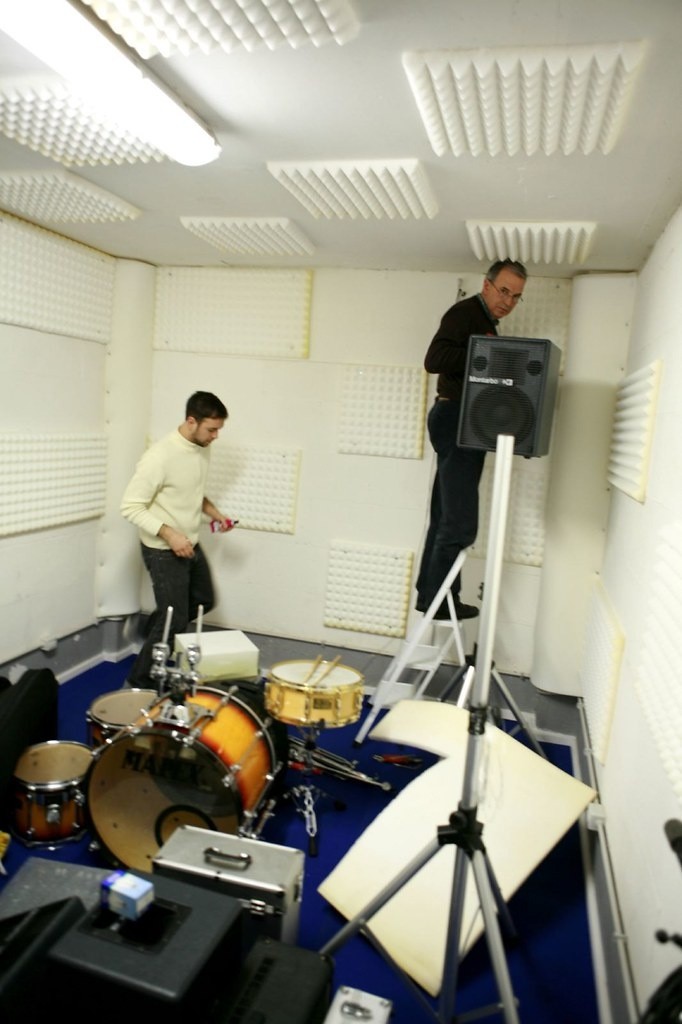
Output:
[435,638,553,764]
[319,434,554,1024]
[276,728,348,856]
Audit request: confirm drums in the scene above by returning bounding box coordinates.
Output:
[9,738,94,849]
[87,687,153,748]
[83,688,275,876]
[264,659,365,727]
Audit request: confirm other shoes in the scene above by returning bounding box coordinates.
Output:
[416,601,479,620]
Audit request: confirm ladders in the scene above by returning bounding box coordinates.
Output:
[354,547,467,746]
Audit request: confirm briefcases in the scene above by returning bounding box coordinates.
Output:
[152,825,305,945]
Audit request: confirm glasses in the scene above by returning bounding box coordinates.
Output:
[489,279,523,305]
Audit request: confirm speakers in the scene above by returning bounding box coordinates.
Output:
[457,335,566,460]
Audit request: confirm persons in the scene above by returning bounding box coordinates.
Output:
[120,389,235,694]
[416,259,528,622]
